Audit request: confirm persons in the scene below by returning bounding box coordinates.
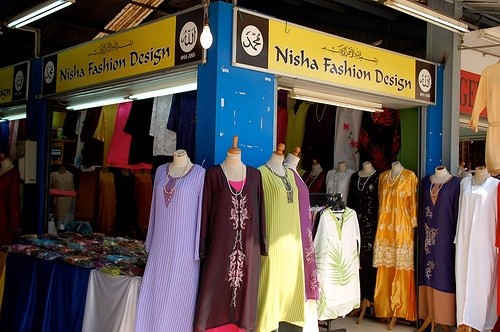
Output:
[49,150,320,332]
[0,151,19,244]
[323,157,500,332]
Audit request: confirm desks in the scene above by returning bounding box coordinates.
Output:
[0,245,144,332]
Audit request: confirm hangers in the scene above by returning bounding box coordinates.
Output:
[309,192,346,214]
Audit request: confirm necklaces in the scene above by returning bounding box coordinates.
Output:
[308,168,323,189]
[266,162,294,192]
[429,174,451,195]
[163,159,191,194]
[468,174,489,193]
[284,164,307,187]
[221,162,246,196]
[387,166,404,186]
[332,169,348,182]
[357,169,375,191]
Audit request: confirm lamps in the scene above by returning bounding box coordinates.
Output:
[4,0,76,30]
[287,87,384,113]
[65,79,197,111]
[477,30,500,46]
[0,104,27,124]
[384,0,471,36]
